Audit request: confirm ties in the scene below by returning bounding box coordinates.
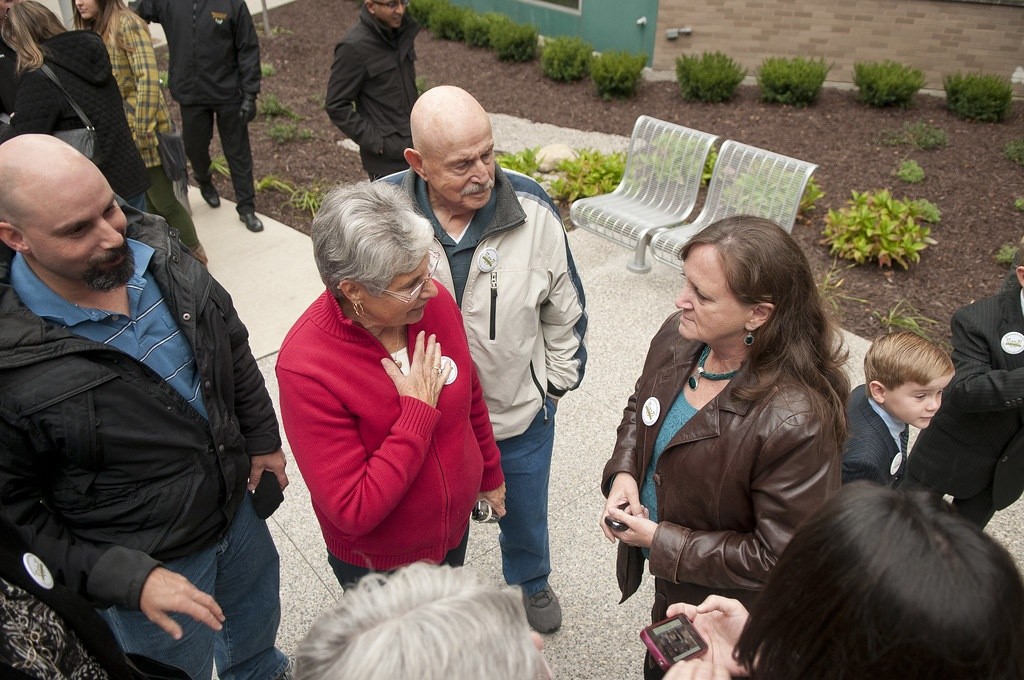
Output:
[899,422,909,476]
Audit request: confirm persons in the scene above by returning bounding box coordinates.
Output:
[1,133,291,680]
[602,215,848,680]
[291,560,554,680]
[0,574,191,679]
[0,0,263,263]
[658,479,1024,680]
[900,236,1024,531]
[373,86,588,635]
[275,182,506,592]
[840,331,955,490]
[661,627,690,657]
[324,1,419,181]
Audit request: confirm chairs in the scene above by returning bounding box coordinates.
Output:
[570,115,818,280]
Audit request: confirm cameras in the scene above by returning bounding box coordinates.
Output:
[472,500,500,523]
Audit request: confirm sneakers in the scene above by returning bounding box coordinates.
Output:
[522,581,562,633]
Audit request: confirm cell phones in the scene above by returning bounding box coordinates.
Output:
[605,502,633,530]
[251,470,285,520]
[640,613,708,673]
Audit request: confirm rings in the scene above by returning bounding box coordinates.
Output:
[433,366,441,372]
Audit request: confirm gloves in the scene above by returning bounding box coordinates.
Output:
[238,99,257,125]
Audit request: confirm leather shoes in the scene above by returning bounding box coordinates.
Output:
[198,181,221,208]
[240,212,263,232]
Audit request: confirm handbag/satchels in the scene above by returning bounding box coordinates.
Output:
[39,64,102,166]
[154,130,189,199]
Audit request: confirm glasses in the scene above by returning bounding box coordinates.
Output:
[372,0,410,9]
[337,249,441,305]
[4,7,12,23]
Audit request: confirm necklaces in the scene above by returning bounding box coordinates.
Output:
[377,327,402,368]
[688,346,736,390]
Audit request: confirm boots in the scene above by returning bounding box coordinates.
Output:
[187,245,208,270]
